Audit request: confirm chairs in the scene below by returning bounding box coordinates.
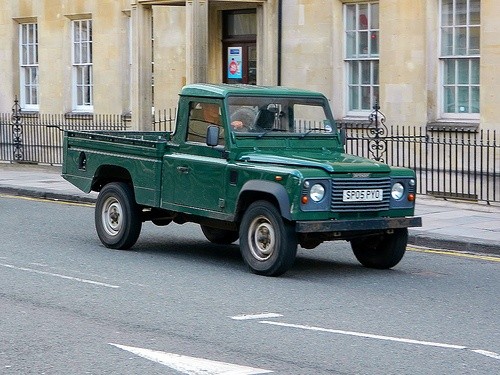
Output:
[230,108,257,131]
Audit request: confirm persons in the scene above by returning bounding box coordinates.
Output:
[188,103,243,147]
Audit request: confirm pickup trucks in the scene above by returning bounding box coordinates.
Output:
[60,83,422,277]
